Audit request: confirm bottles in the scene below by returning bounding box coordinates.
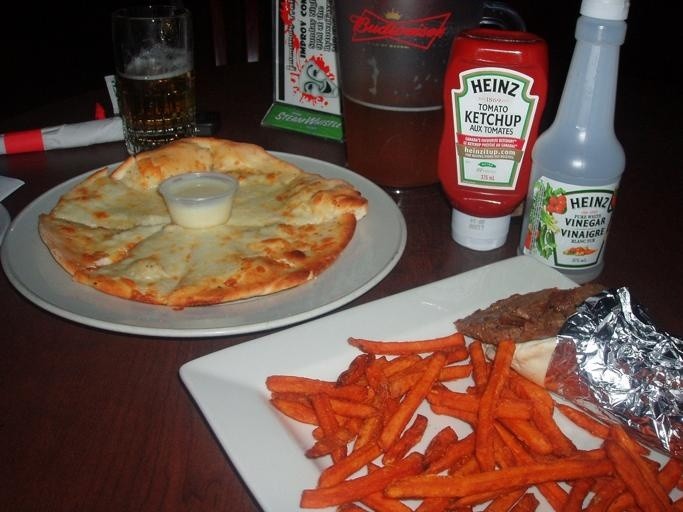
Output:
[337,0,472,113]
[523,1,632,288]
[435,28,551,253]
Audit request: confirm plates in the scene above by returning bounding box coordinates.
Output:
[179,254,683,512]
[5,147,409,336]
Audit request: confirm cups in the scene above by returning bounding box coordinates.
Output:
[106,2,198,158]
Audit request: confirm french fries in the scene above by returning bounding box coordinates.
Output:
[264,332,683,512]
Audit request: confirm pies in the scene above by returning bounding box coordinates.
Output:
[39,136,369,308]
